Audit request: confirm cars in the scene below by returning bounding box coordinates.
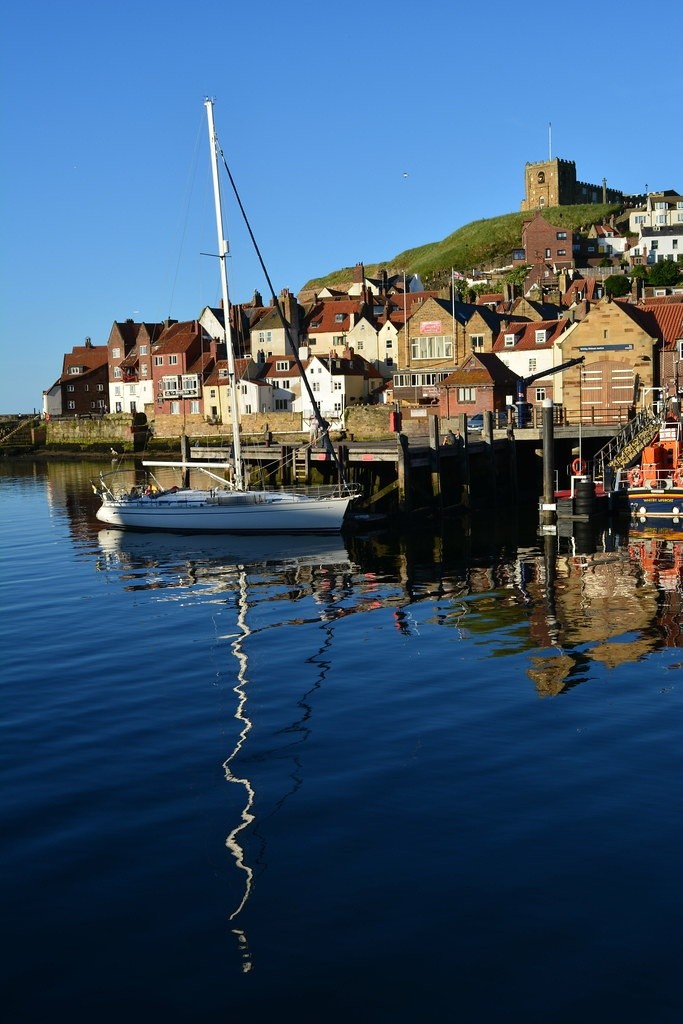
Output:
[467,411,505,434]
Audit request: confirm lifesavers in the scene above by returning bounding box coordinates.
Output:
[571,459,587,474]
[673,468,683,488]
[628,469,642,486]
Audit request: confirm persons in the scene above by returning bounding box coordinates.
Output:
[455,431,464,447]
[310,416,319,448]
[507,420,514,442]
[443,430,455,446]
[319,418,330,448]
[130,487,141,500]
[145,485,153,496]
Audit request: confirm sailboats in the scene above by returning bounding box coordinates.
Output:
[84,93,365,533]
